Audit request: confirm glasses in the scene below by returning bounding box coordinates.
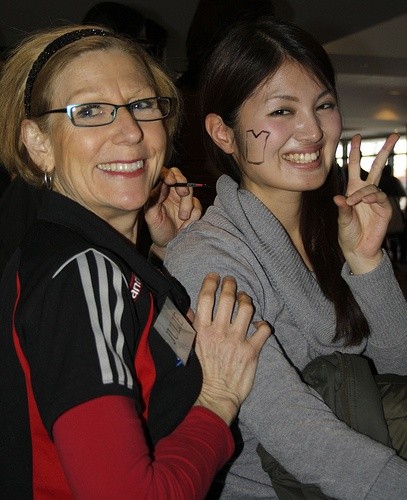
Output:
[37,96,172,127]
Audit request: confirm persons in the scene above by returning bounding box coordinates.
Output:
[0,26,276,499]
[340,150,407,271]
[163,19,407,499]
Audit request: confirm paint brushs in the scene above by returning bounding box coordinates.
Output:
[155,182,206,187]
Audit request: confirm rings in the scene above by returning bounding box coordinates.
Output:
[377,187,382,192]
